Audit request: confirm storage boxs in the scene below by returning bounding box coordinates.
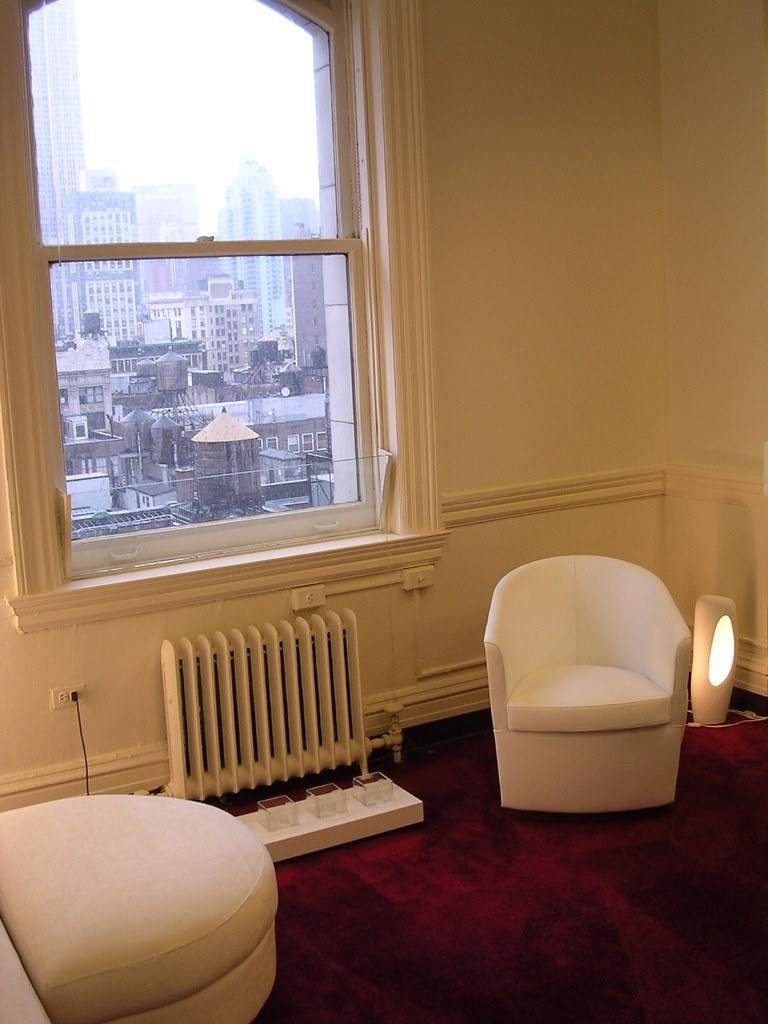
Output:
[352,771,395,806]
[257,795,300,834]
[305,783,348,818]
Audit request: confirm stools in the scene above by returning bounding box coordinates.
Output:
[0,796,281,1024]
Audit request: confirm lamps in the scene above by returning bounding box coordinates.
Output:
[688,594,741,726]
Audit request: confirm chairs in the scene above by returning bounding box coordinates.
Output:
[483,554,692,813]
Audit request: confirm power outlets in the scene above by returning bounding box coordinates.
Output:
[291,582,328,611]
[51,683,87,709]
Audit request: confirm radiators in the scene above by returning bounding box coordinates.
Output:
[135,607,405,801]
